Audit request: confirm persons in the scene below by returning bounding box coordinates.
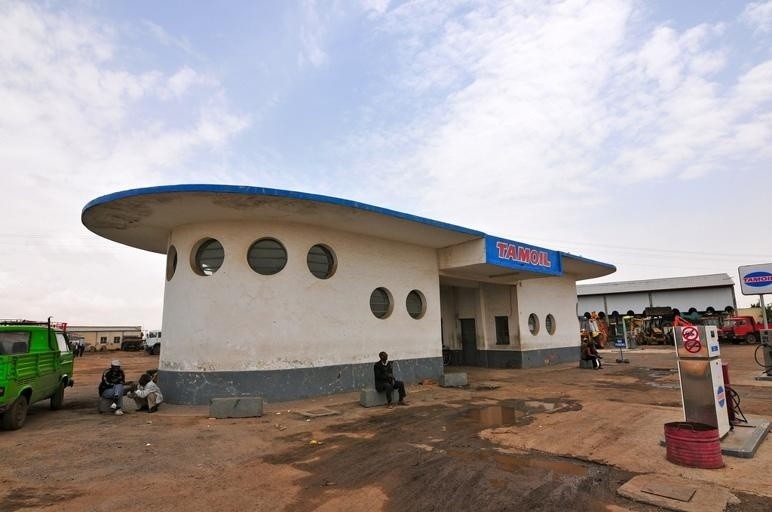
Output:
[70,341,85,358]
[99,360,126,415]
[582,341,604,370]
[127,374,163,412]
[372,351,409,409]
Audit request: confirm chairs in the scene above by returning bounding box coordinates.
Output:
[0,340,27,355]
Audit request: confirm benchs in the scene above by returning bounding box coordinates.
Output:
[208,397,264,419]
[439,372,468,388]
[360,387,400,408]
[97,396,144,412]
[579,358,594,369]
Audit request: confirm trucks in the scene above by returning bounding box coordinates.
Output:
[0,318,75,430]
[721,315,771,345]
[700,315,728,343]
[142,329,162,355]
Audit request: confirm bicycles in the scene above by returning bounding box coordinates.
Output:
[442,345,452,366]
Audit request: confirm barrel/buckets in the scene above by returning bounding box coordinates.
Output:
[663,422,722,470]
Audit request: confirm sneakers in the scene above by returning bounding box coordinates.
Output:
[398,400,410,405]
[110,403,116,409]
[114,409,124,416]
[136,405,158,413]
[387,404,392,409]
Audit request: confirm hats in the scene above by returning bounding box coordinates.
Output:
[111,360,121,366]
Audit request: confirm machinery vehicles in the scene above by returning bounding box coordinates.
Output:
[580,310,609,349]
[630,313,691,346]
